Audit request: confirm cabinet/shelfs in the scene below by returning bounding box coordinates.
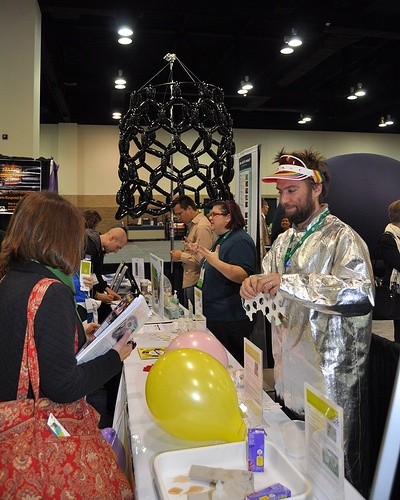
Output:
[0,156,49,233]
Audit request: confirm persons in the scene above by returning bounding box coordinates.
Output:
[240,146,375,492]
[0,191,136,429]
[378,200,400,342]
[170,195,219,315]
[181,200,258,368]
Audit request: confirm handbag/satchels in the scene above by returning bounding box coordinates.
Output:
[0,278,133,500]
[371,231,400,321]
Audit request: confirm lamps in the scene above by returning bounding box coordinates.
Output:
[287,28,302,47]
[297,112,311,125]
[347,83,366,100]
[237,81,248,96]
[378,114,394,128]
[113,69,126,90]
[280,35,294,55]
[117,15,134,44]
[242,76,253,90]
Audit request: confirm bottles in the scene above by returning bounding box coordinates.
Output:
[140,279,148,292]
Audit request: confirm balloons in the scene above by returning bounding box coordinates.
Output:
[102,427,125,472]
[145,348,246,443]
[166,330,229,368]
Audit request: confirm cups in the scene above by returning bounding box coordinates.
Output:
[280,420,305,458]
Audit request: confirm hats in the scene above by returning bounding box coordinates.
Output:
[261,154,326,183]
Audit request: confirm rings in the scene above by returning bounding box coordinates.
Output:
[128,341,136,350]
[269,282,274,287]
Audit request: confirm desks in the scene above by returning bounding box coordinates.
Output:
[128,225,166,241]
[115,321,366,500]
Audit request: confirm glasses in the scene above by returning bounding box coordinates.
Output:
[207,212,227,218]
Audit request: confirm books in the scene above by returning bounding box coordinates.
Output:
[76,291,152,366]
